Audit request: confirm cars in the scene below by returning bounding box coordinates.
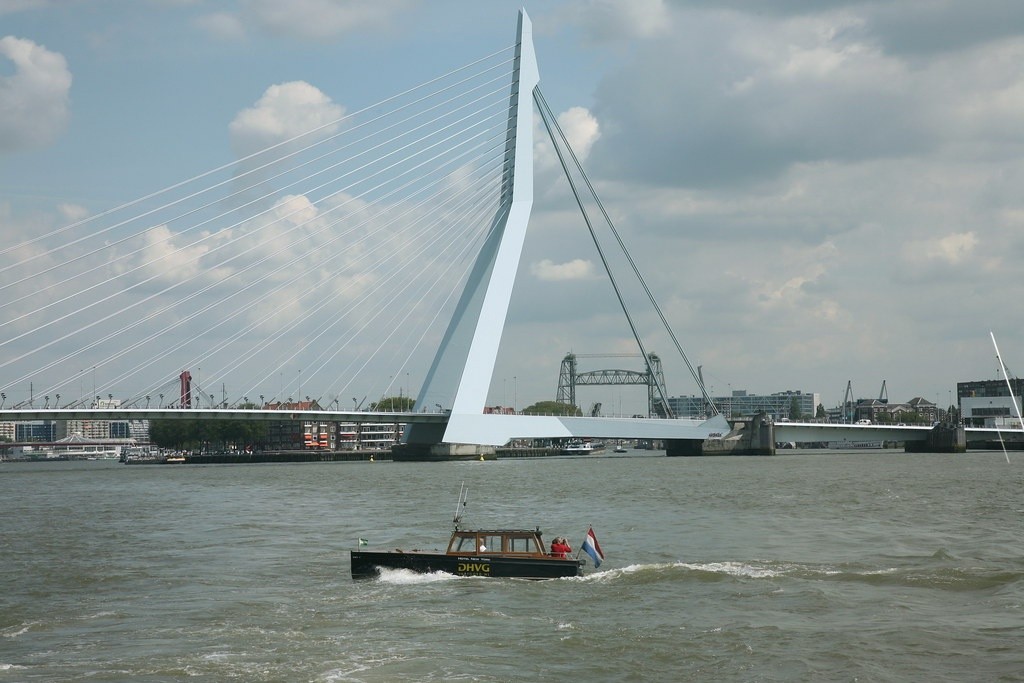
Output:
[856,419,871,426]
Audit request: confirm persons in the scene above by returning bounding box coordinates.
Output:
[551,537,571,560]
[472,538,486,552]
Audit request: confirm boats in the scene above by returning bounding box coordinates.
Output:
[612,439,628,453]
[348,480,587,584]
[561,439,595,456]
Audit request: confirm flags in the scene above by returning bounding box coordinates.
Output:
[582,527,604,569]
[360,538,368,546]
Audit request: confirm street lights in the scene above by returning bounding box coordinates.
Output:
[406,372,410,413]
[728,383,732,420]
[81,370,84,407]
[513,376,518,415]
[389,375,394,412]
[198,367,202,408]
[948,390,953,422]
[503,378,507,414]
[297,369,302,410]
[92,366,97,408]
